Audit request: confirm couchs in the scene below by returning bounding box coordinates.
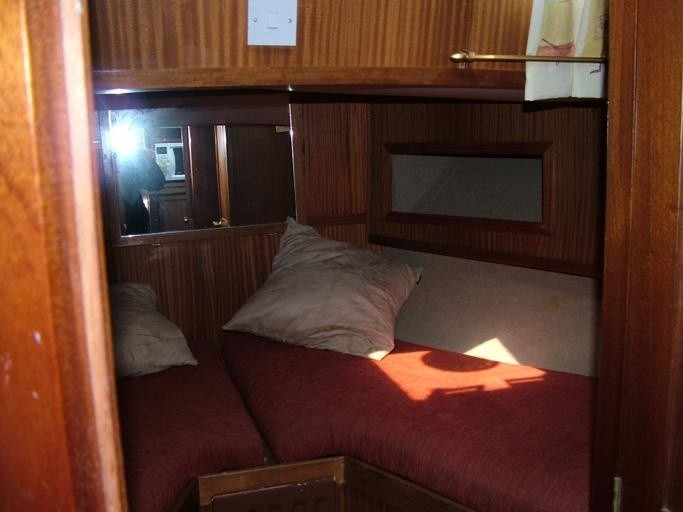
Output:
[216,239,594,512]
[123,352,266,512]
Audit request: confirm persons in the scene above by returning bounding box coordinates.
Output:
[121,147,165,234]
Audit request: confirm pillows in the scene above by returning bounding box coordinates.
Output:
[218,217,425,362]
[107,283,197,381]
